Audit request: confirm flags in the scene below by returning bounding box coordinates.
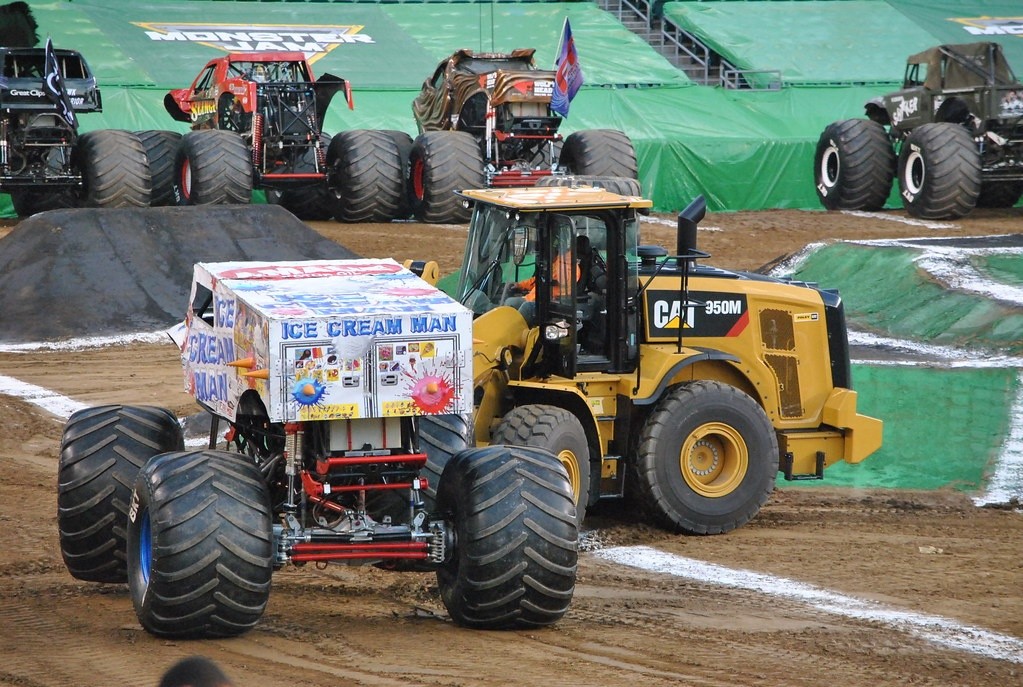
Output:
[45,38,80,129]
[550,19,584,119]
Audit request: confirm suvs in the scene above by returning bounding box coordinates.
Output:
[53,257,579,629]
[0,42,153,217]
[139,51,402,228]
[810,42,1023,221]
[379,48,641,226]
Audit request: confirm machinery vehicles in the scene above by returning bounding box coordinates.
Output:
[394,188,883,535]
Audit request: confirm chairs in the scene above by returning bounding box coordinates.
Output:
[556,235,606,318]
[245,65,268,83]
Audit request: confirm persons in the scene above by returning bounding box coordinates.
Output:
[505,229,581,326]
